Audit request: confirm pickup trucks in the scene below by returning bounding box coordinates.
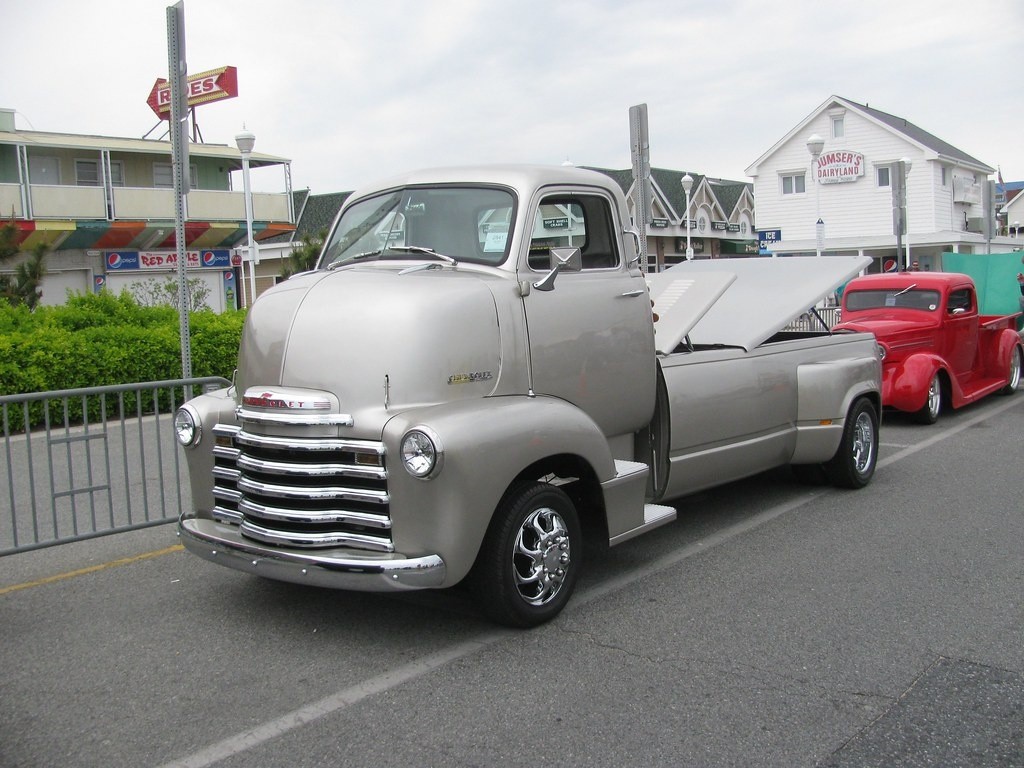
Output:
[830,271,1024,425]
[169,163,887,630]
